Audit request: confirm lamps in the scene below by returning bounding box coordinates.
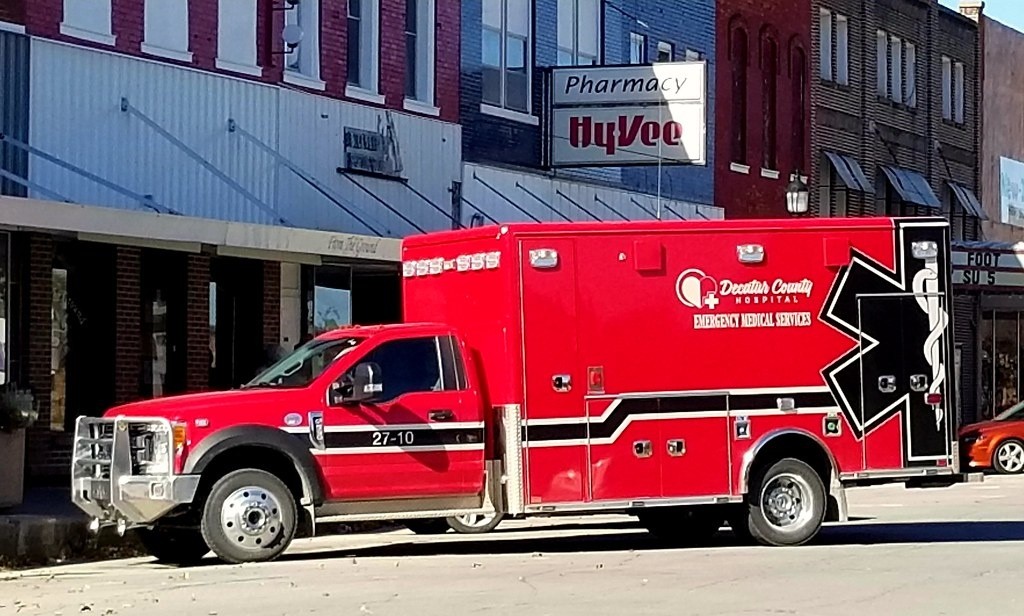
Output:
[268,25,304,55]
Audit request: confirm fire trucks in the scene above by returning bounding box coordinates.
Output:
[67,219,986,570]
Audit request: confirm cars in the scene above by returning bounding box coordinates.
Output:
[959,399,1024,477]
